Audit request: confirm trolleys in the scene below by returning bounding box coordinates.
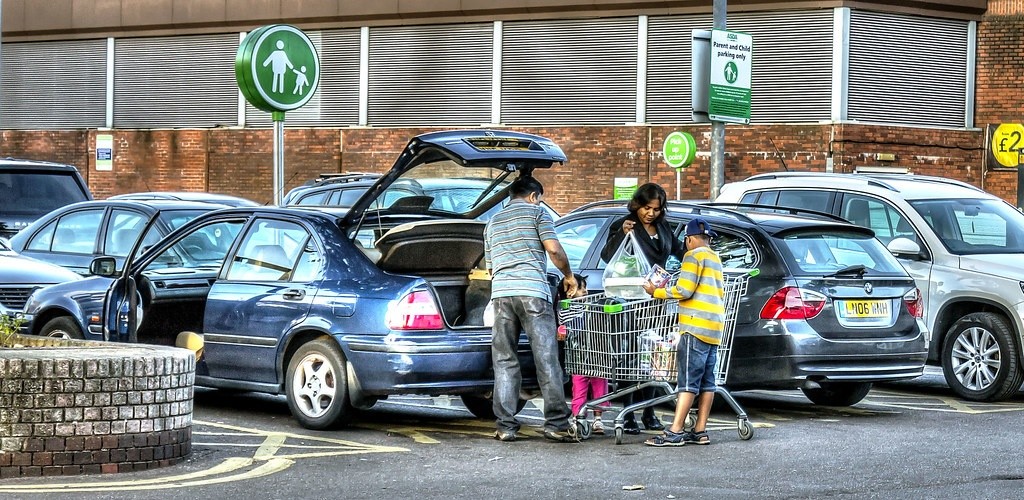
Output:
[558,269,759,444]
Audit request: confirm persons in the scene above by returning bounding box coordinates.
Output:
[553,274,611,435]
[600,183,684,435]
[483,177,584,442]
[642,218,726,447]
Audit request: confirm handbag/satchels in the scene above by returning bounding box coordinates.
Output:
[602,231,651,300]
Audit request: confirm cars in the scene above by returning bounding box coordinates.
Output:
[0,236,83,319]
[106,191,262,207]
[9,199,320,280]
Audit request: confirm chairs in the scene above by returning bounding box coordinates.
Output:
[112,228,137,255]
[50,228,75,251]
[894,215,915,241]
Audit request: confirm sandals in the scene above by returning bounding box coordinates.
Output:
[644,429,686,447]
[685,429,710,445]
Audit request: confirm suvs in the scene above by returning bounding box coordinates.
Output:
[285,170,559,221]
[716,171,1024,403]
[25,129,568,431]
[0,158,96,240]
[547,199,929,406]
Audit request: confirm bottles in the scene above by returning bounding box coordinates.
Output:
[641,340,653,374]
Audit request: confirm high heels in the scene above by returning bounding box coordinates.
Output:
[641,415,665,430]
[623,419,640,434]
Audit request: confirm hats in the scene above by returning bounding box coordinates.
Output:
[682,218,717,251]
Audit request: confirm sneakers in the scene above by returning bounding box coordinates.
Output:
[544,429,582,442]
[493,430,516,441]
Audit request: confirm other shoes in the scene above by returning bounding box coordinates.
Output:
[591,417,604,431]
